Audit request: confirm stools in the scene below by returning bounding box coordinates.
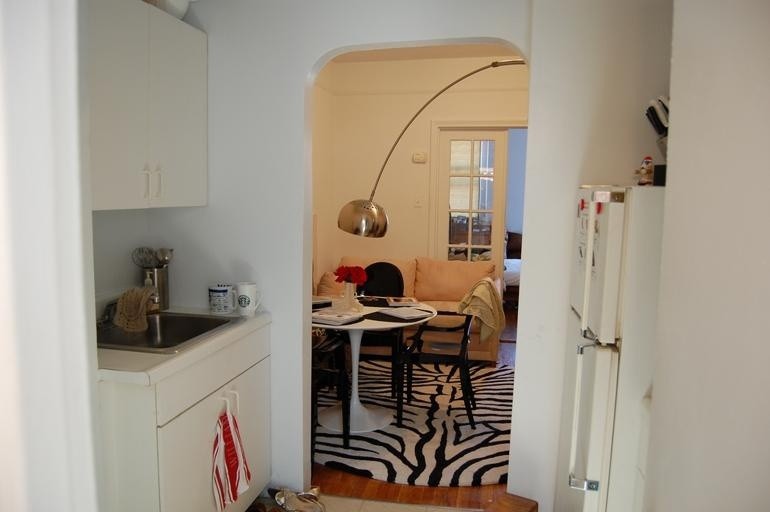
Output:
[312,350,351,450]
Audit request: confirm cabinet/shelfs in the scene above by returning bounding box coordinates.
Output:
[78,0,209,213]
[98,322,272,512]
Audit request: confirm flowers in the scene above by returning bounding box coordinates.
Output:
[333,265,369,285]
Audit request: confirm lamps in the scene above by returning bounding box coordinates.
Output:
[336,57,525,239]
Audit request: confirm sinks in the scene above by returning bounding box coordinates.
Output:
[97,312,247,355]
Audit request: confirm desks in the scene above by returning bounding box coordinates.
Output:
[313,300,438,433]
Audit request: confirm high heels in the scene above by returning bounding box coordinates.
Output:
[267,485,326,512]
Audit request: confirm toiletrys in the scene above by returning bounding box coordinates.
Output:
[145,271,159,316]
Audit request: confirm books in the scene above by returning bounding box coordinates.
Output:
[312,309,363,325]
[386,296,419,306]
[376,308,433,319]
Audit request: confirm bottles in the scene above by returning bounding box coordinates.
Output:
[340,283,359,314]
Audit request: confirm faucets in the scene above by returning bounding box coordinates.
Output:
[103,294,159,323]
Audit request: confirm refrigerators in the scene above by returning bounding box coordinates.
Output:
[553,184,667,511]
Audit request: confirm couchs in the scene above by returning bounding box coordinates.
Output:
[317,255,505,367]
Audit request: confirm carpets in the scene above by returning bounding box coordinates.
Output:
[311,359,514,487]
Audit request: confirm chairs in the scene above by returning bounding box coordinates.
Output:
[337,261,492,430]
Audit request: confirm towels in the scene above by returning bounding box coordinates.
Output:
[210,412,253,512]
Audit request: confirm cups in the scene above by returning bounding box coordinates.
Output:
[208,283,261,317]
